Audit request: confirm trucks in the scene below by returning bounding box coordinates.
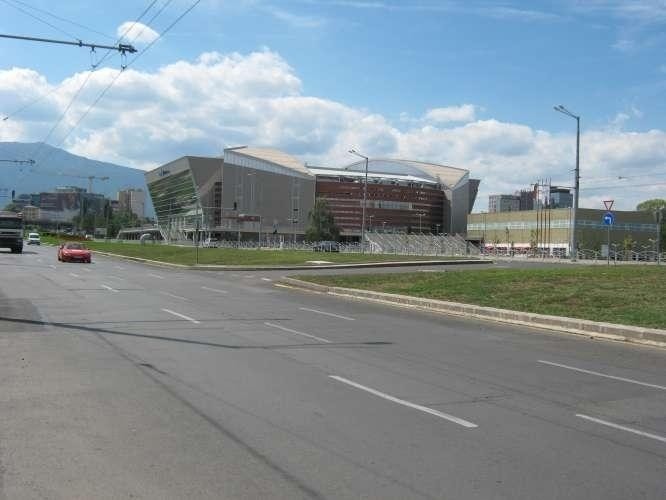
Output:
[0,210,24,254]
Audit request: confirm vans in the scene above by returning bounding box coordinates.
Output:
[27,232,41,246]
[202,237,217,249]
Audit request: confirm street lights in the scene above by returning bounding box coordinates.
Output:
[436,224,441,237]
[369,215,375,233]
[160,194,172,245]
[348,149,369,255]
[381,221,387,233]
[415,213,426,235]
[191,195,199,264]
[552,104,581,261]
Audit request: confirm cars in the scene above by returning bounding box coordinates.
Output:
[58,241,92,264]
[314,240,340,254]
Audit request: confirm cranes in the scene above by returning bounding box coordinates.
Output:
[56,171,110,192]
